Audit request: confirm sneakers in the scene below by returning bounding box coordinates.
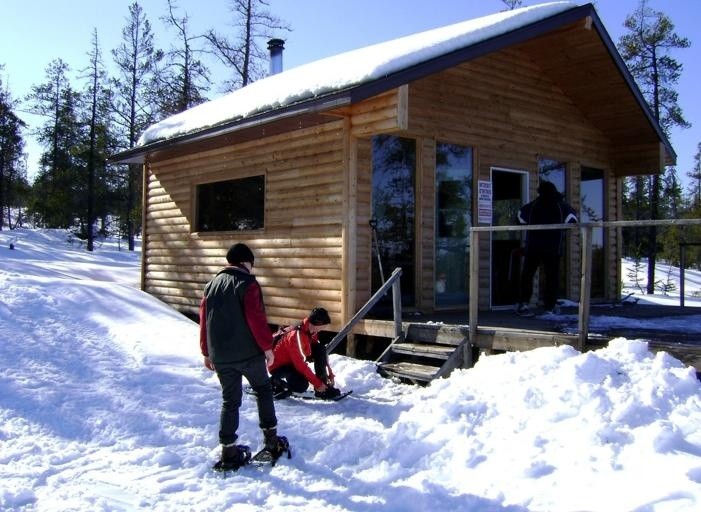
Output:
[517,304,528,317]
[552,304,561,314]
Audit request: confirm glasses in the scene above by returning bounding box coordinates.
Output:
[251,262,254,267]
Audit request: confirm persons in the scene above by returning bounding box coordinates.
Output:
[200,242,290,467]
[505,180,579,318]
[267,302,342,399]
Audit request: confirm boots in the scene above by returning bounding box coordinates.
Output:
[222,445,244,463]
[314,384,340,397]
[263,428,284,453]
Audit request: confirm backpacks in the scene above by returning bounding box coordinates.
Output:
[273,327,282,347]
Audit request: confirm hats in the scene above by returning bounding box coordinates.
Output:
[538,182,557,194]
[308,308,330,325]
[227,242,254,263]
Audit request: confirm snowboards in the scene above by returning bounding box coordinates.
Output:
[243,383,353,401]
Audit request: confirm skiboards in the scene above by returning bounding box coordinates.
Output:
[213,436,288,472]
